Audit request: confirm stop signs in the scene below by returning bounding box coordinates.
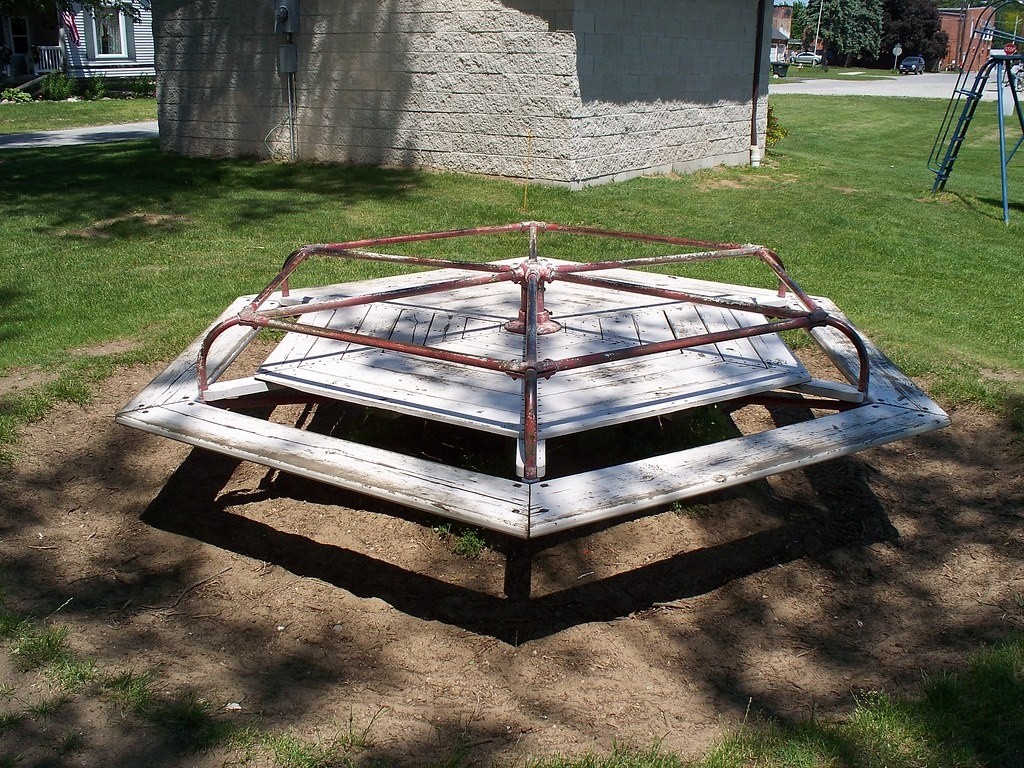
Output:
[1004,43,1017,55]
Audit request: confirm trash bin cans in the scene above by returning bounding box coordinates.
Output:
[771,63,790,77]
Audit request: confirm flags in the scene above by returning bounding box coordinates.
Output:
[61,1,81,46]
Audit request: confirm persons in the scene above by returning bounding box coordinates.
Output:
[1005,60,1024,91]
[790,50,798,63]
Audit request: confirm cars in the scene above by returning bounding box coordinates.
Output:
[791,52,822,65]
[899,57,926,75]
